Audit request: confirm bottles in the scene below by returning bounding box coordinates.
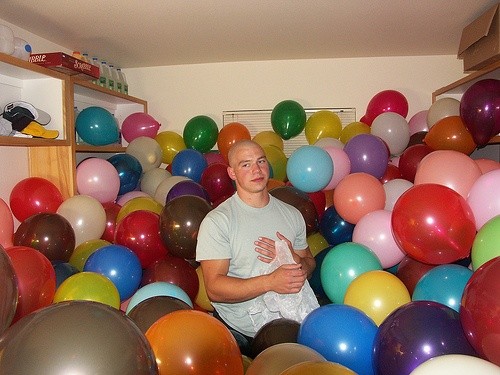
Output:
[74,107,82,143]
[117,69,128,94]
[13,37,31,62]
[72,52,86,62]
[0,24,15,54]
[92,57,100,85]
[109,65,121,92]
[83,54,90,63]
[100,62,111,88]
[111,114,122,147]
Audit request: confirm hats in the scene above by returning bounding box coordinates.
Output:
[3,100,59,139]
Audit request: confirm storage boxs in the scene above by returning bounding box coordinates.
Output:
[29,53,100,82]
[457,2,500,72]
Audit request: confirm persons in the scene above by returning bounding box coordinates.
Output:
[195,140,322,342]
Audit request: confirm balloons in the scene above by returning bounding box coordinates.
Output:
[0,78,500,375]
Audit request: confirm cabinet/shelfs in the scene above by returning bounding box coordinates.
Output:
[0,53,148,198]
